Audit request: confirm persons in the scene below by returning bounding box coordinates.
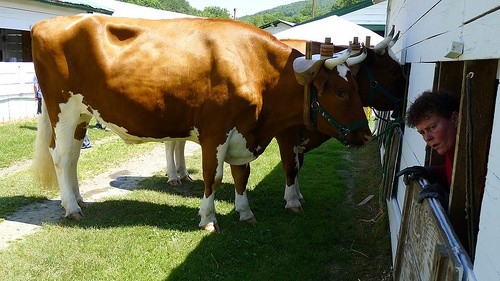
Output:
[396,90,460,203]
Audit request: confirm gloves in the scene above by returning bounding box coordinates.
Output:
[397,165,429,185]
[419,184,439,200]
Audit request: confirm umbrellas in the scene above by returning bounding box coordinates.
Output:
[270,14,391,48]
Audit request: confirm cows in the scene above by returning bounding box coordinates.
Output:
[30,12,373,233]
[165,24,409,216]
